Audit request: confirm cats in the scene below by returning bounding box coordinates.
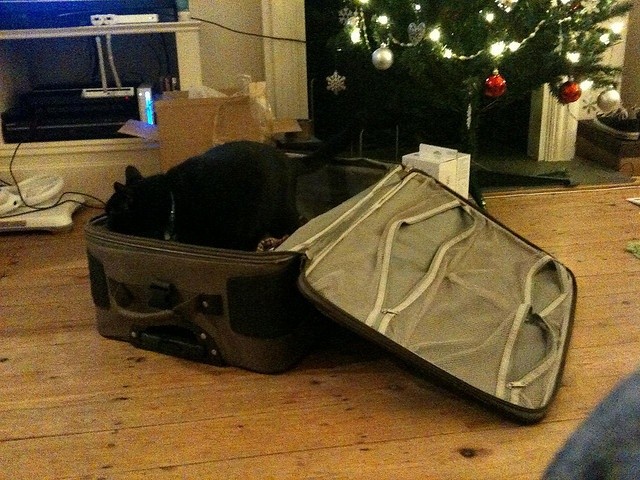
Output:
[103,127,349,252]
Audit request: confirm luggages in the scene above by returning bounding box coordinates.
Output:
[83,152,576,423]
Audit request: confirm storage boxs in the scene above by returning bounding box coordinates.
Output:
[117,74,303,173]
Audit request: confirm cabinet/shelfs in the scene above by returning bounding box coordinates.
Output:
[0,20,203,205]
